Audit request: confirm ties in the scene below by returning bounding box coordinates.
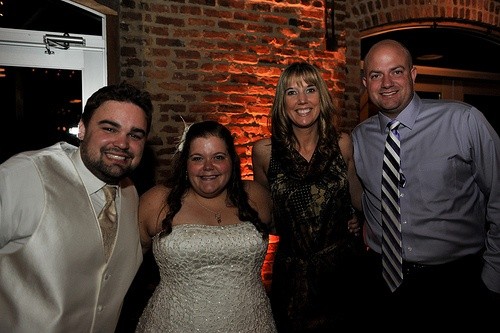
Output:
[381,120,404,293]
[96,186,118,264]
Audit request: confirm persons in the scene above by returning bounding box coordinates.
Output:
[0,85,153,333]
[138,120,360,333]
[349,40,500,333]
[251,62,364,333]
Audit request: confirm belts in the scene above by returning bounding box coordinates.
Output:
[402,261,430,274]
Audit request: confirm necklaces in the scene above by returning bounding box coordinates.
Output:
[194,192,227,223]
